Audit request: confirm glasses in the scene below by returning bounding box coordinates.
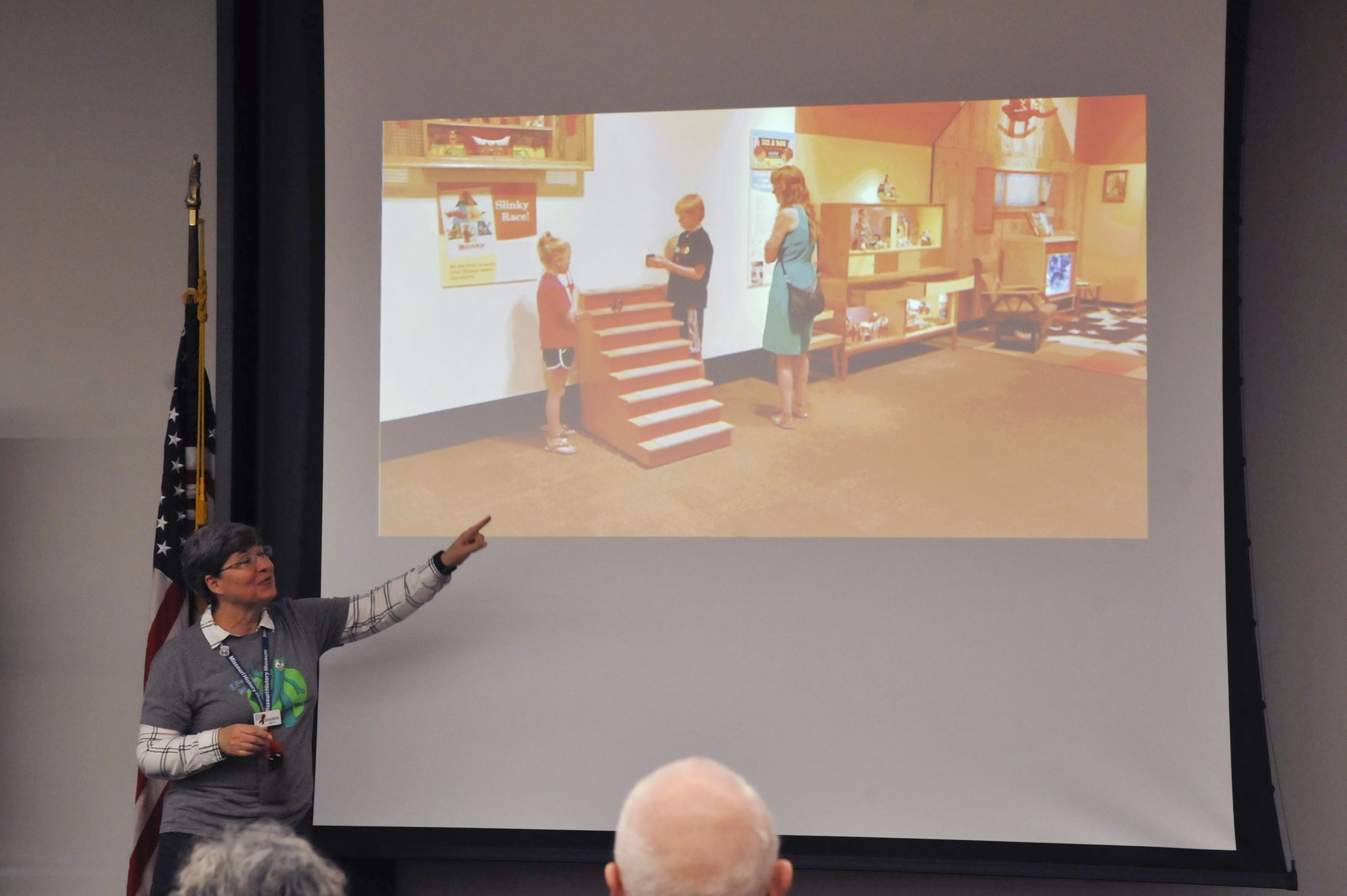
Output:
[212,545,274,577]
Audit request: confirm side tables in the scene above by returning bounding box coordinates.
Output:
[1073,281,1104,314]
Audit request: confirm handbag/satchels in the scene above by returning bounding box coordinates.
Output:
[785,275,825,333]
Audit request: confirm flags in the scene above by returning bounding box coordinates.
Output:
[119,151,218,896]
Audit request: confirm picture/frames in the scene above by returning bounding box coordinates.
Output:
[1102,170,1127,203]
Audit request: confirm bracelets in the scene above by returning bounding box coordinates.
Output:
[432,548,457,576]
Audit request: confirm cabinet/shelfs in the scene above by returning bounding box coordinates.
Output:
[815,201,967,381]
[382,115,594,196]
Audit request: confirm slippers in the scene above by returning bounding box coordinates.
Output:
[559,424,578,438]
[772,411,794,430]
[543,436,578,456]
[791,406,809,418]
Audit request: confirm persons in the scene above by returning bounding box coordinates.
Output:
[534,229,582,455]
[167,815,350,895]
[132,513,496,895]
[645,192,715,380]
[761,164,822,430]
[601,755,793,896]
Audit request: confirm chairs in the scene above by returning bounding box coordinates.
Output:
[972,255,1058,340]
